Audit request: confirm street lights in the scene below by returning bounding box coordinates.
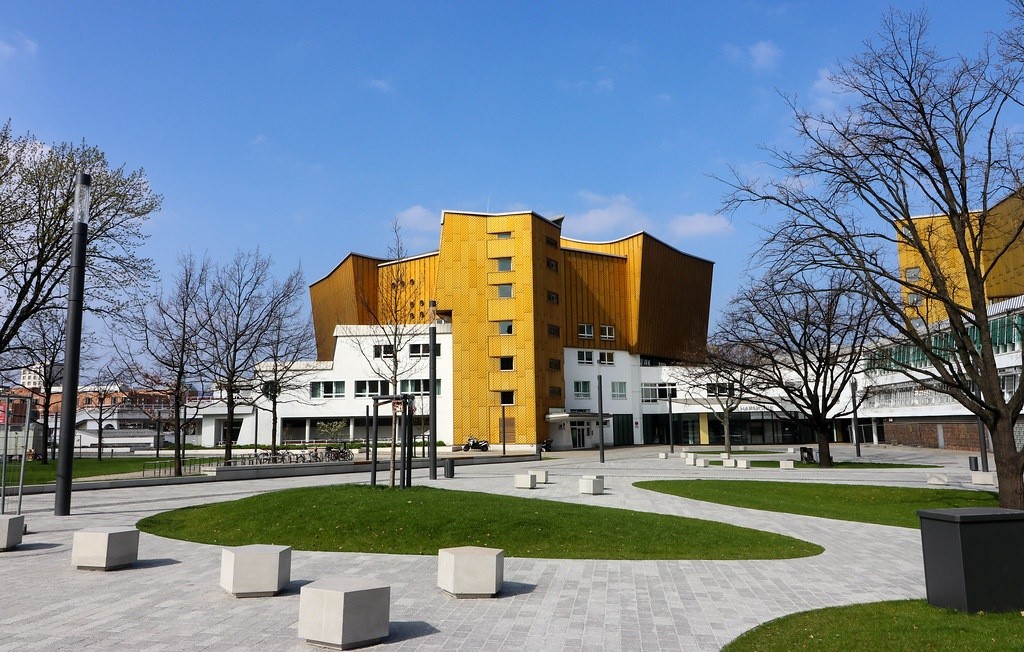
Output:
[54,172,92,517]
[848,360,861,457]
[427,301,438,479]
[970,352,988,472]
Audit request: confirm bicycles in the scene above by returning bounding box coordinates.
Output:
[258,444,353,463]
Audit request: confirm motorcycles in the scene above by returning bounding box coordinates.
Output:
[463,437,489,451]
